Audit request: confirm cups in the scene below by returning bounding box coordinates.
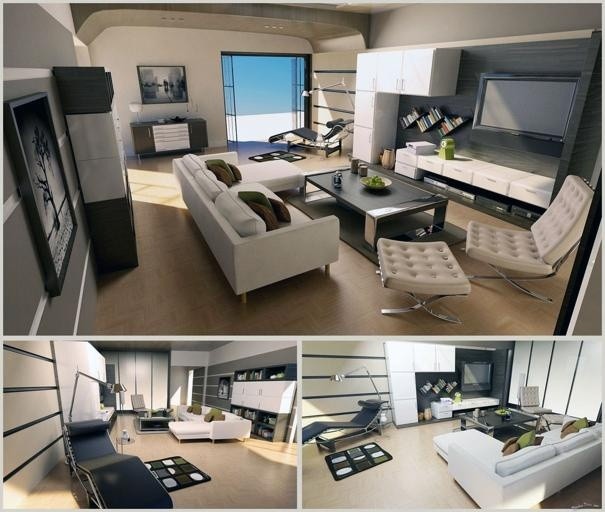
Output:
[501,409,513,422]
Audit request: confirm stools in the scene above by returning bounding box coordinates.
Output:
[371,237,473,325]
[539,412,567,431]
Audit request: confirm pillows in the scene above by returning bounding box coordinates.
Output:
[237,189,274,213]
[560,424,581,438]
[226,162,244,183]
[205,158,238,183]
[573,416,591,430]
[244,200,278,232]
[517,429,537,448]
[534,435,545,445]
[187,404,226,423]
[501,436,519,452]
[561,419,578,432]
[268,195,292,224]
[502,442,520,457]
[205,164,234,188]
[587,420,597,426]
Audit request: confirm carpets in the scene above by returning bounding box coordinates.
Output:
[283,184,470,266]
[247,149,307,163]
[325,440,394,483]
[143,454,212,491]
[131,417,170,435]
[446,423,548,443]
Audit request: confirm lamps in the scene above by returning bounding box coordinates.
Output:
[66,367,128,423]
[128,104,144,125]
[328,365,392,427]
[301,80,355,112]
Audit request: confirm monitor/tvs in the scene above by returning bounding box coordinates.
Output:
[461,361,492,391]
[470,71,580,141]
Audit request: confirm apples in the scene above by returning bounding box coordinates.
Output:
[365,175,385,186]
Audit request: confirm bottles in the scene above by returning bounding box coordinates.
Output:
[351,158,367,176]
[332,171,343,189]
[455,392,462,404]
[143,411,152,419]
[472,409,489,417]
[379,148,395,169]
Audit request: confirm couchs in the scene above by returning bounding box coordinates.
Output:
[167,404,252,445]
[432,420,602,510]
[170,150,344,307]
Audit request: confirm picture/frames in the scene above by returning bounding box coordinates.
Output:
[216,376,231,401]
[4,91,79,300]
[136,65,189,106]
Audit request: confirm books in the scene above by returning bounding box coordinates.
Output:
[232,406,275,438]
[236,370,262,380]
[400,105,463,137]
[419,379,458,395]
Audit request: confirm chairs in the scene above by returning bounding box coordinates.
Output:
[60,415,175,508]
[301,398,389,454]
[130,393,152,416]
[456,171,595,301]
[517,384,554,428]
[267,117,354,160]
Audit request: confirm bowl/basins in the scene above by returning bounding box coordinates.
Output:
[172,117,186,122]
[360,177,394,190]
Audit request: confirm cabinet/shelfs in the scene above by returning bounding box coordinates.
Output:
[52,63,116,116]
[233,362,297,381]
[383,340,419,426]
[414,342,457,374]
[102,407,118,433]
[62,101,128,206]
[417,377,461,396]
[430,395,501,422]
[230,380,297,415]
[376,47,465,99]
[229,404,291,443]
[352,51,402,166]
[82,189,142,276]
[398,103,472,138]
[53,340,108,423]
[128,117,210,160]
[393,147,556,230]
